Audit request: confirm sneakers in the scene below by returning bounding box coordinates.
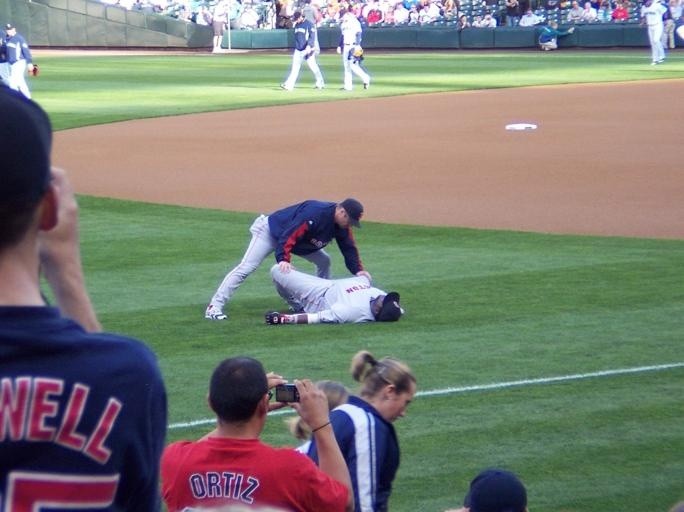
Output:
[205,304,227,320]
[289,306,305,312]
[279,76,370,90]
[651,58,665,65]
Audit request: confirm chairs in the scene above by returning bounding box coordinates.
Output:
[356,0,644,30]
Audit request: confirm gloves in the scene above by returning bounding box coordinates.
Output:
[265,311,285,325]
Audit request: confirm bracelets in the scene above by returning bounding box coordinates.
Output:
[312,421,331,434]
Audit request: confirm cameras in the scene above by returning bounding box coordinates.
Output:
[275,383,300,403]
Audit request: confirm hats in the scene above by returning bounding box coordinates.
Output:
[376,292,400,321]
[463,470,527,511]
[292,8,347,21]
[5,23,14,30]
[343,198,363,228]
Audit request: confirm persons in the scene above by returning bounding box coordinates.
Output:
[124,0,684,31]
[205,198,371,321]
[441,468,530,512]
[0,23,34,101]
[0,29,10,80]
[336,8,371,90]
[641,0,676,65]
[212,1,228,52]
[280,12,324,91]
[1,80,170,511]
[160,355,354,512]
[307,350,417,511]
[284,380,351,454]
[539,23,573,51]
[266,264,403,326]
